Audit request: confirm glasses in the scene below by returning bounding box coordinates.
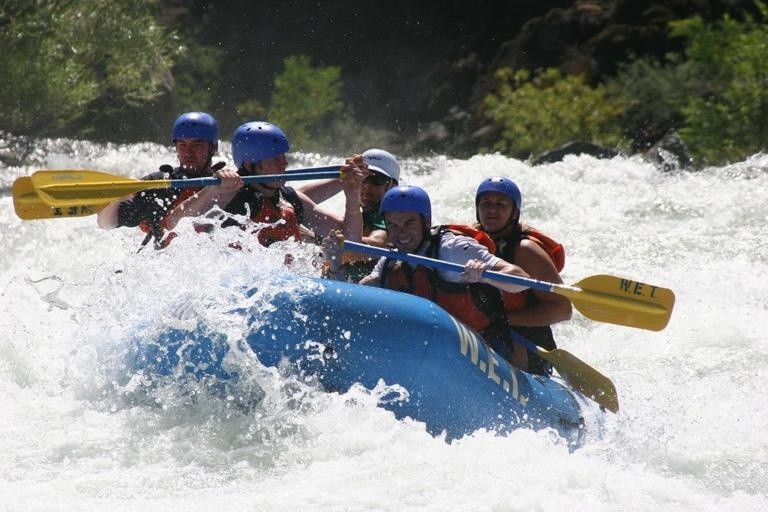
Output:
[362,174,389,186]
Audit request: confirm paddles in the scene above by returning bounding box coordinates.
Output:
[12,166,344,221]
[511,331,620,412]
[344,240,675,331]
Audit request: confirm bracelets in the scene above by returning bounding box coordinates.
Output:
[344,204,363,214]
[328,263,343,274]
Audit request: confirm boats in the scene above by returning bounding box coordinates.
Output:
[132,273,607,454]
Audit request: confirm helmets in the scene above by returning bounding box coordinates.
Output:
[476,176,521,218]
[362,148,400,185]
[380,185,431,227]
[232,120,289,169]
[172,112,217,146]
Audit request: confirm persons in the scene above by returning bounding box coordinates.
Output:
[321,186,531,371]
[326,150,401,282]
[466,177,571,377]
[99,112,369,239]
[164,122,362,255]
